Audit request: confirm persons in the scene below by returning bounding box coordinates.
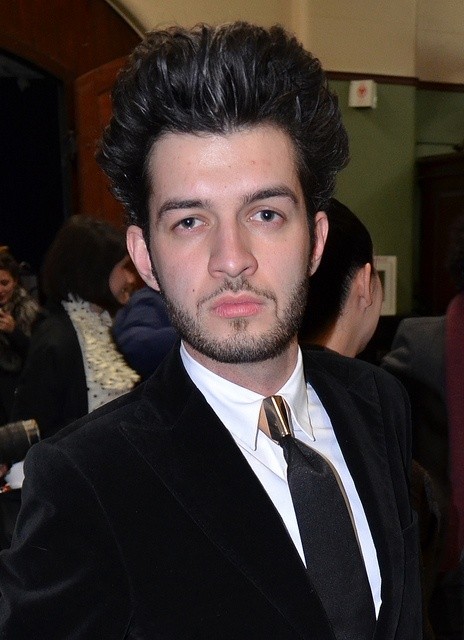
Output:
[0,22,423,640]
[303,197,383,358]
[9,214,144,440]
[0,251,49,426]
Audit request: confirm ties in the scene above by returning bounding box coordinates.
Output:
[258,395,376,640]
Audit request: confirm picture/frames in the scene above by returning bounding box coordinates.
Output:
[373,256,398,316]
